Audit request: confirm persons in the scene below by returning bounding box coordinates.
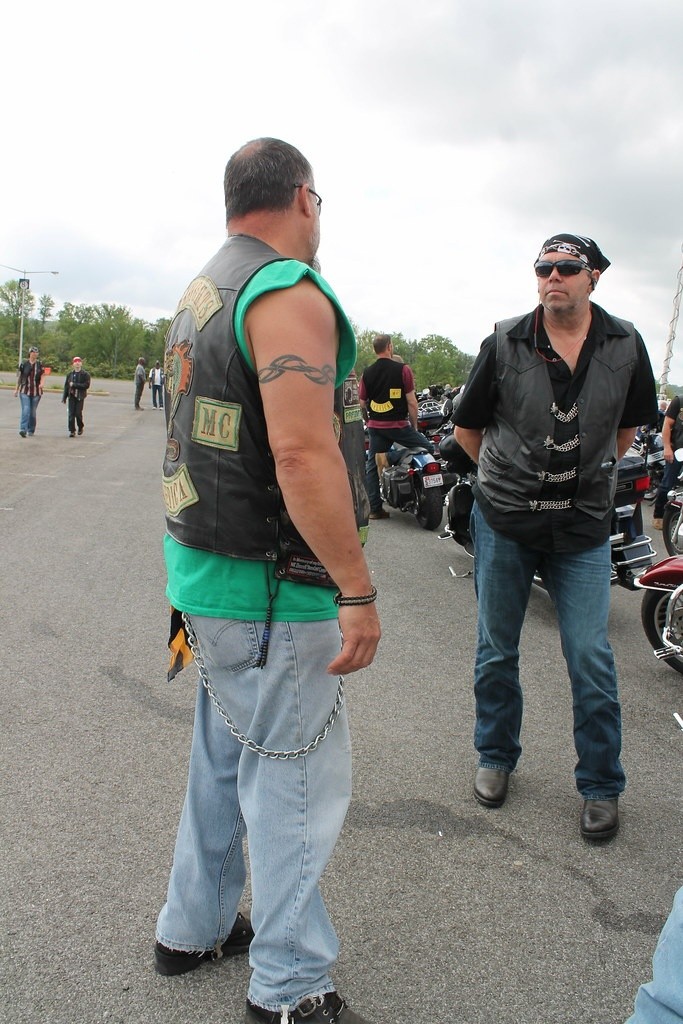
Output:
[148,360,165,410]
[451,233,659,838]
[134,357,146,410]
[636,395,683,529]
[60,357,90,436]
[357,333,434,519]
[152,136,381,1024]
[14,347,44,438]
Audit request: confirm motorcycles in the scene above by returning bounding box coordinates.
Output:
[357,381,683,681]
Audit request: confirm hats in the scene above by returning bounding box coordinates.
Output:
[28,347,39,354]
[73,356,81,362]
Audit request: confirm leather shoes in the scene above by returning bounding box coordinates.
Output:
[473,767,508,806]
[580,798,619,838]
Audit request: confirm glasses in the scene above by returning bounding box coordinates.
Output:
[295,183,322,214]
[534,259,592,277]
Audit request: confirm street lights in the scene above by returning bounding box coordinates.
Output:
[0,264,59,377]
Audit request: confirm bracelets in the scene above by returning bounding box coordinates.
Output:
[334,585,378,605]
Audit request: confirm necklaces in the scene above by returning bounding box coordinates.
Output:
[531,302,591,362]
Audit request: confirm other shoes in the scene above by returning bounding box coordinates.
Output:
[152,407,157,410]
[154,910,255,975]
[135,407,144,411]
[245,990,376,1024]
[70,432,76,437]
[78,428,83,435]
[653,518,663,529]
[368,508,390,519]
[19,430,26,437]
[28,431,35,436]
[159,407,163,410]
